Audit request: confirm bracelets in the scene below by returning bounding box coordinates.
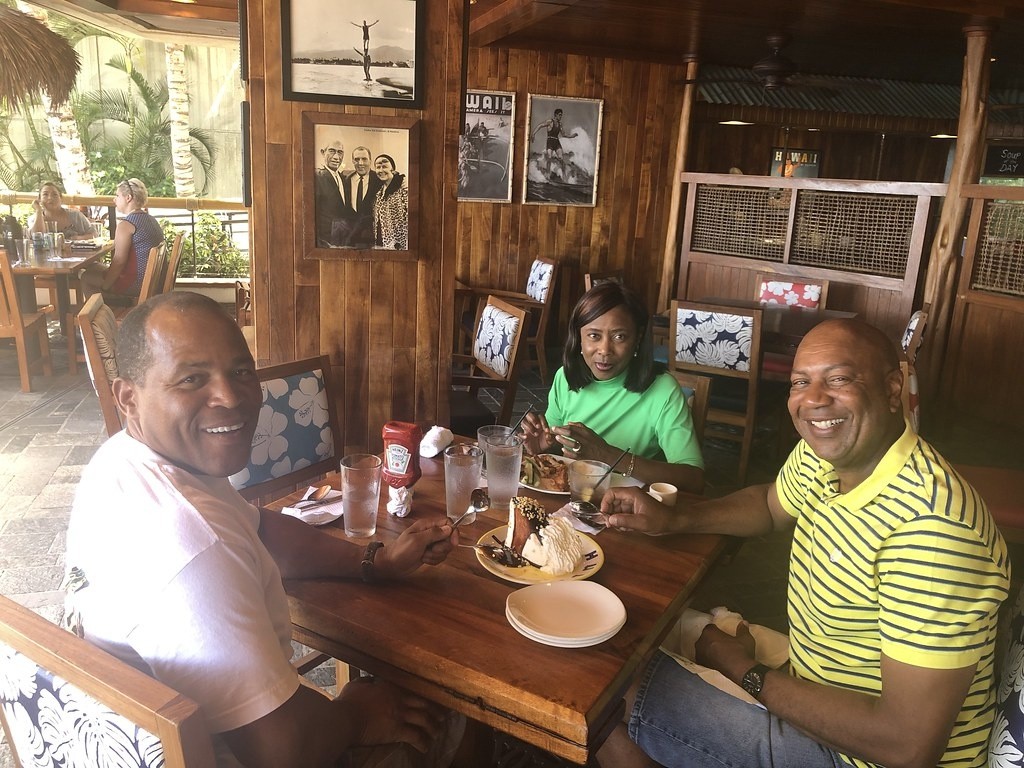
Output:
[626,452,635,477]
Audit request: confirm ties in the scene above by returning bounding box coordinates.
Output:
[356,176,364,212]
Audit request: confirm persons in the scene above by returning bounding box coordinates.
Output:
[518,280,705,494]
[350,20,380,79]
[595,318,1011,768]
[16,182,97,337]
[80,179,165,302]
[532,107,580,184]
[315,141,408,251]
[60,291,468,768]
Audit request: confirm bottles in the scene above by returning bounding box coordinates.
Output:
[382,421,422,517]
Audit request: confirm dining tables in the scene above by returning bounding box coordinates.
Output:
[256,430,741,768]
[651,296,867,469]
[9,239,115,374]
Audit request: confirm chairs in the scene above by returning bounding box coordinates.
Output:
[0,233,1023,768]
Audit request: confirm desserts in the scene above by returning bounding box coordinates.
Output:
[504,496,585,573]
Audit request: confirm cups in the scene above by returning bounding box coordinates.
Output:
[63,240,72,257]
[485,434,523,510]
[340,454,382,538]
[45,222,57,233]
[102,230,110,244]
[15,239,30,266]
[47,235,62,260]
[54,233,64,248]
[477,425,515,476]
[650,483,677,507]
[444,445,484,526]
[33,232,43,251]
[569,460,612,523]
[646,492,663,503]
[44,233,53,250]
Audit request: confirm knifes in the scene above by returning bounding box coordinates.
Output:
[299,495,342,512]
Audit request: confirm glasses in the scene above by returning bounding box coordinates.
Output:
[123,179,133,203]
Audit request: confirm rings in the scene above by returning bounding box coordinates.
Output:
[573,441,583,453]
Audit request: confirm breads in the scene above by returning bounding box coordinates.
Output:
[538,454,569,491]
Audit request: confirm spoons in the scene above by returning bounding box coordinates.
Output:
[571,501,625,534]
[288,485,331,508]
[451,488,490,531]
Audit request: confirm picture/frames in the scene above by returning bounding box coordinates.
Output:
[281,0,426,110]
[302,111,421,262]
[768,147,823,180]
[522,94,604,208]
[457,89,517,204]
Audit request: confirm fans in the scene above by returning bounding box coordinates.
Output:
[671,34,881,91]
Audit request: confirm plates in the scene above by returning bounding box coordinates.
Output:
[505,581,627,648]
[475,525,604,585]
[519,454,577,494]
[288,490,344,525]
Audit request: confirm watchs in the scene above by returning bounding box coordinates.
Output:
[742,663,773,699]
[360,541,384,585]
